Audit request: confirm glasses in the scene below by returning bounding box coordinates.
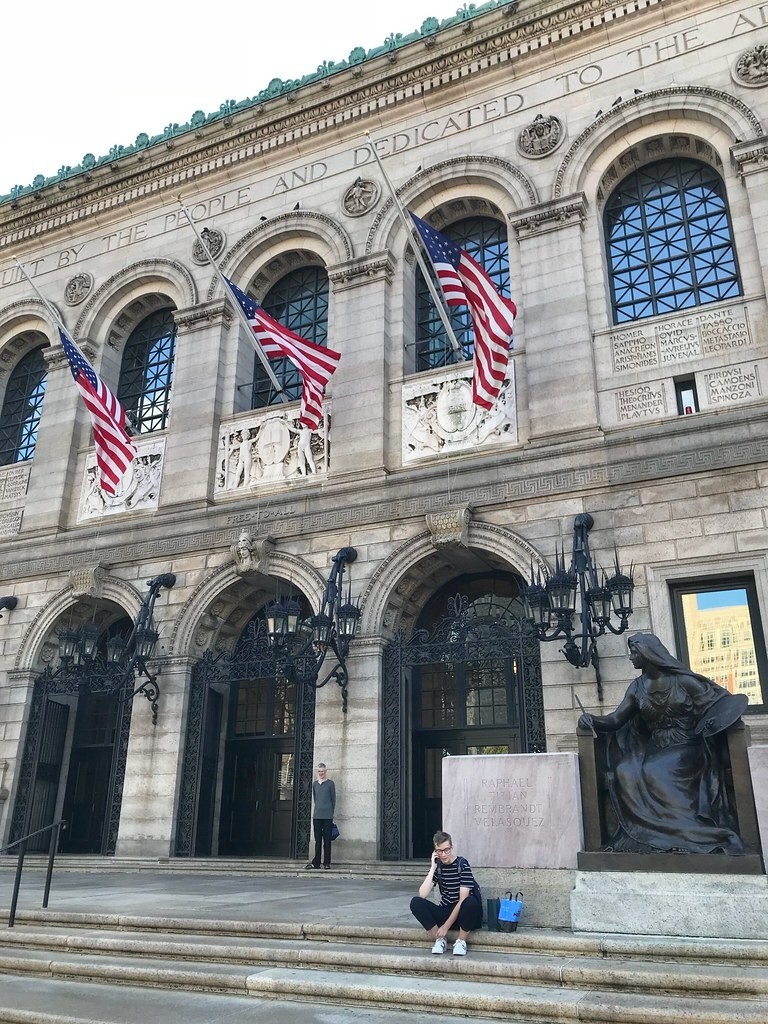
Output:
[435,847,452,854]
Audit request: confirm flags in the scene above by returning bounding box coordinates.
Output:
[406,207,518,412]
[221,272,341,431]
[58,326,136,498]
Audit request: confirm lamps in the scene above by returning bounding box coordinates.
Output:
[50,573,176,725]
[514,513,635,701]
[263,547,362,712]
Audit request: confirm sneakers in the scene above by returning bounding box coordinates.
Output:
[453,939,467,955]
[432,937,447,953]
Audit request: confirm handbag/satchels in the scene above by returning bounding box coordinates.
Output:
[497,892,523,933]
[487,897,501,931]
[331,824,340,841]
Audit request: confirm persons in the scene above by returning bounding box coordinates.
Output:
[231,425,261,489]
[578,633,749,855]
[282,417,325,476]
[346,182,372,211]
[304,763,336,869]
[238,532,255,572]
[409,831,483,958]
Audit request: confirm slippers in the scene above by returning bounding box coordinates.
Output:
[302,864,321,869]
[324,866,330,869]
